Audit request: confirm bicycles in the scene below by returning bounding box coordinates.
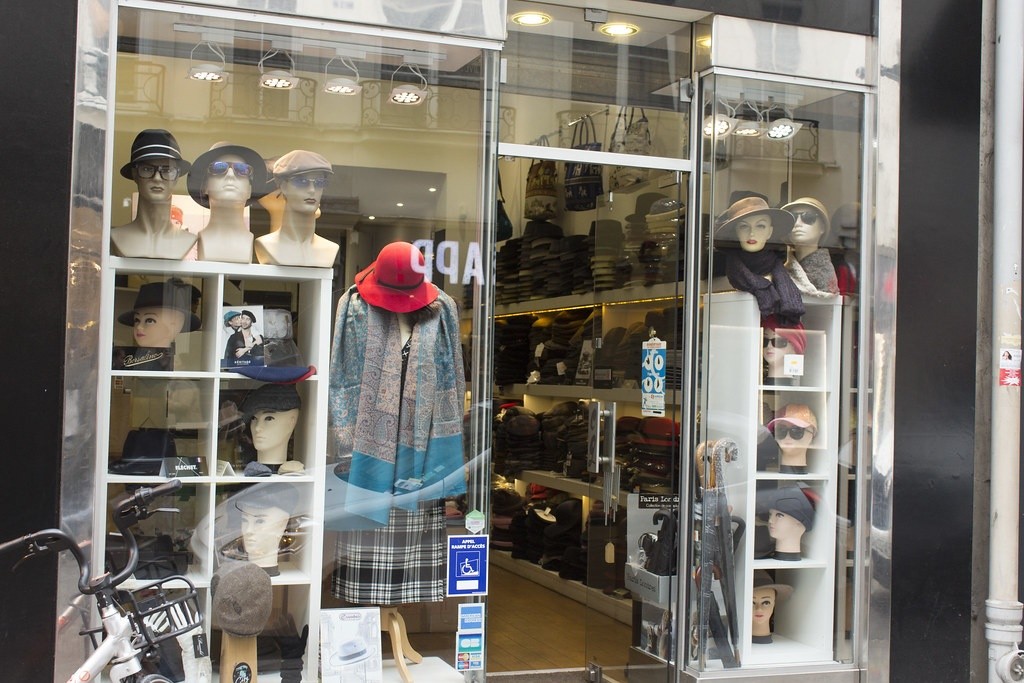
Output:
[0,478,202,683]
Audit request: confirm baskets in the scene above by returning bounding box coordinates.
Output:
[68,556,179,629]
[78,575,203,661]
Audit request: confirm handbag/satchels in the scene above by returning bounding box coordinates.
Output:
[522,108,651,214]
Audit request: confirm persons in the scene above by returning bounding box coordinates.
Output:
[778,197,830,260]
[119,283,202,347]
[762,314,807,378]
[236,482,299,568]
[223,311,263,359]
[187,142,266,264]
[326,242,465,682]
[713,197,794,253]
[752,570,792,635]
[254,150,340,268]
[110,130,198,261]
[769,487,815,552]
[766,403,817,466]
[241,384,301,470]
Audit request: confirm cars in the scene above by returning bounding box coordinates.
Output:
[187,426,490,590]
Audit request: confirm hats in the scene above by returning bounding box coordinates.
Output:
[102,385,308,579]
[354,242,438,312]
[759,487,816,532]
[753,569,792,609]
[712,195,795,252]
[121,281,200,336]
[768,405,817,436]
[187,141,270,207]
[459,193,683,581]
[272,150,334,185]
[213,565,273,635]
[240,310,256,324]
[120,129,192,181]
[780,198,831,248]
[224,311,241,328]
[762,312,805,355]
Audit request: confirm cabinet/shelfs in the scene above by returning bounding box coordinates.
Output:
[459,278,858,670]
[96,257,335,683]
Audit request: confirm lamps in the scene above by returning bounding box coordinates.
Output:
[257,40,305,89]
[185,33,234,82]
[765,97,803,141]
[323,47,367,95]
[732,92,768,137]
[386,55,434,106]
[703,92,741,141]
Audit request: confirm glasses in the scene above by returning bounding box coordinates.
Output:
[284,174,328,187]
[772,425,806,441]
[762,337,790,347]
[130,163,182,181]
[790,210,820,226]
[201,162,254,184]
